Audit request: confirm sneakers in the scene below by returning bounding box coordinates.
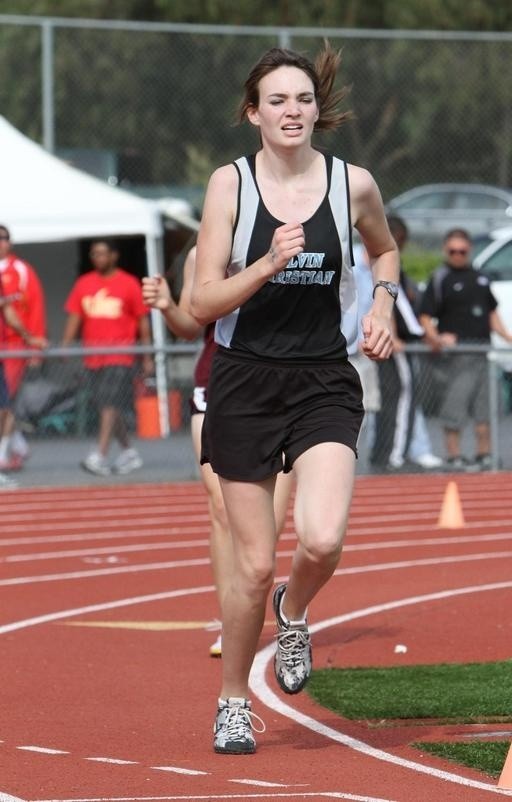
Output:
[207,618,224,658]
[369,453,500,472]
[272,580,313,692]
[80,452,114,478]
[1,430,31,473]
[212,697,259,755]
[111,448,143,475]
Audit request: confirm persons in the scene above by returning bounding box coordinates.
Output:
[186,43,401,756]
[140,218,294,658]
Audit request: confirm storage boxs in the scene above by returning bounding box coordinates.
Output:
[134,388,183,440]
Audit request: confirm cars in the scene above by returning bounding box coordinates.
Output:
[412,220,512,375]
[371,179,512,242]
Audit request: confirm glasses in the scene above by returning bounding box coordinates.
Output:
[1,234,11,242]
[446,247,471,256]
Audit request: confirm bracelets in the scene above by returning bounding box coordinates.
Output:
[372,279,399,299]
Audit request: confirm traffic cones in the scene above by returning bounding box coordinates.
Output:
[494,740,512,790]
[432,478,469,530]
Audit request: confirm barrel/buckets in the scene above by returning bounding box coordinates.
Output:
[137,386,181,438]
